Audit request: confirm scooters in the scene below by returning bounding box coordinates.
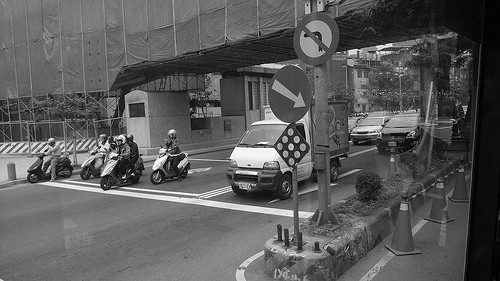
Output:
[100,148,144,191]
[79,146,104,180]
[27,153,73,184]
[149,145,192,185]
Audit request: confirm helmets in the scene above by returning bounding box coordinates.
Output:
[168,129,177,138]
[47,138,55,146]
[100,134,134,144]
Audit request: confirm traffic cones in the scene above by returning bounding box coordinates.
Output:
[384,194,422,256]
[447,166,469,203]
[424,177,455,224]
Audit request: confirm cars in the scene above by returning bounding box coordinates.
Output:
[350,115,392,145]
[347,116,364,141]
[376,114,421,152]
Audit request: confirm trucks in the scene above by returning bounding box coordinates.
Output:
[226,100,351,200]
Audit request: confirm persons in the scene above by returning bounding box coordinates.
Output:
[43,137,61,181]
[98,134,110,162]
[114,134,130,179]
[164,128,183,181]
[126,133,139,172]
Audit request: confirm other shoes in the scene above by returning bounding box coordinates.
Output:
[50,178,55,181]
[178,174,182,181]
[122,173,135,182]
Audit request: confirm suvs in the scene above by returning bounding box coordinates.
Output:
[449,103,465,136]
